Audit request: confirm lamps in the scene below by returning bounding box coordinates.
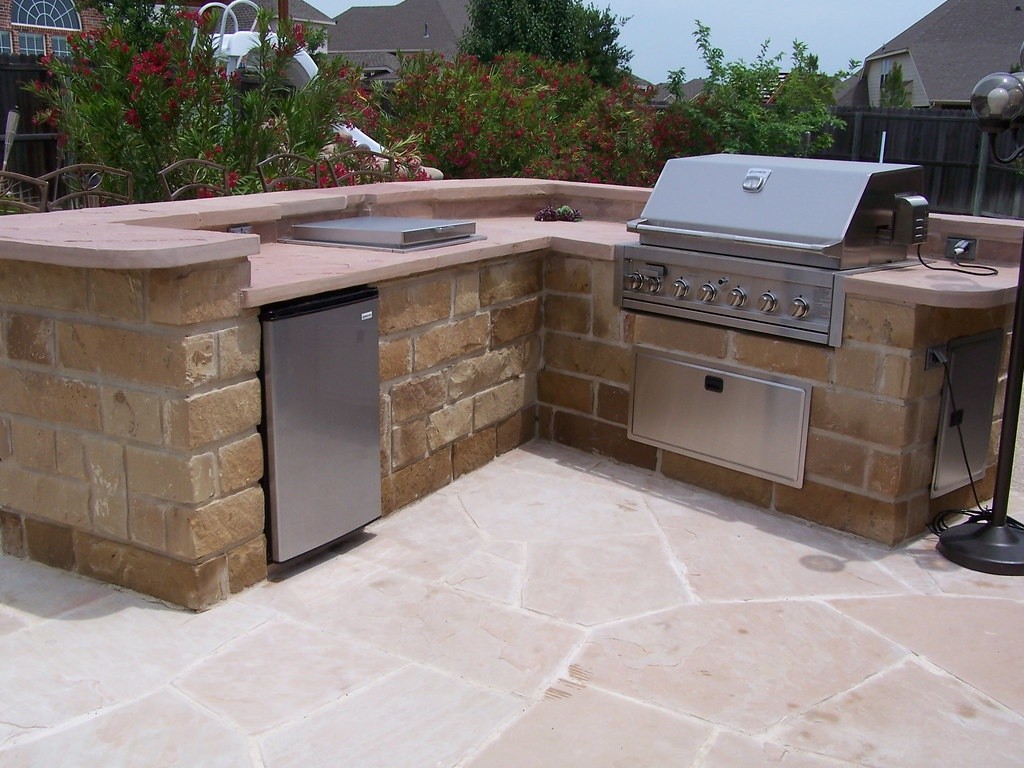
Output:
[970,71,1024,214]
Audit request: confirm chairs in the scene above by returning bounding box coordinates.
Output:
[0,150,395,216]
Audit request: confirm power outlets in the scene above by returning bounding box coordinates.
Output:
[944,237,978,261]
[924,345,948,370]
